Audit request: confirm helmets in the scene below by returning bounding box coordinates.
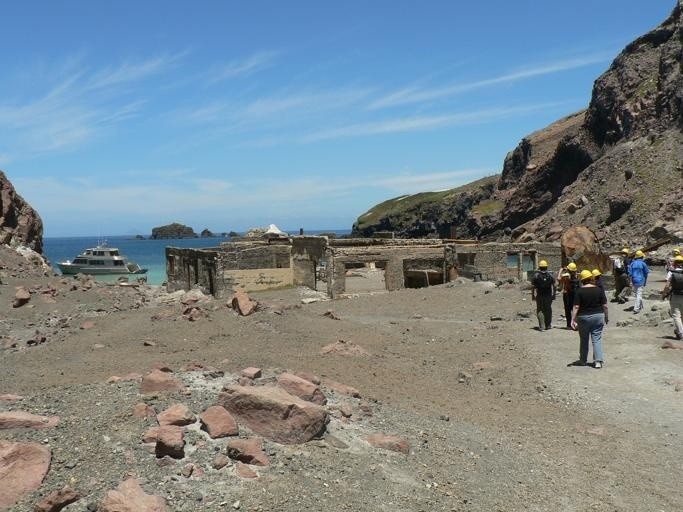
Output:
[634,250,645,259]
[566,262,578,272]
[538,260,549,268]
[591,268,602,278]
[579,269,593,281]
[620,248,630,254]
[672,248,680,255]
[672,255,683,261]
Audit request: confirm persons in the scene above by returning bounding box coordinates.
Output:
[661,256,683,340]
[592,269,605,291]
[531,260,556,331]
[666,249,680,273]
[569,269,609,368]
[613,248,631,303]
[628,251,649,314]
[558,263,579,327]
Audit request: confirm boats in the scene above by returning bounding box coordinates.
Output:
[54,237,146,276]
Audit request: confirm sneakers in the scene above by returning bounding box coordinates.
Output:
[592,360,603,368]
[545,324,552,330]
[673,329,683,339]
[578,360,588,366]
[617,295,626,304]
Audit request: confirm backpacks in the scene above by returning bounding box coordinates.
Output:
[537,273,552,290]
[669,268,683,296]
[568,275,581,294]
